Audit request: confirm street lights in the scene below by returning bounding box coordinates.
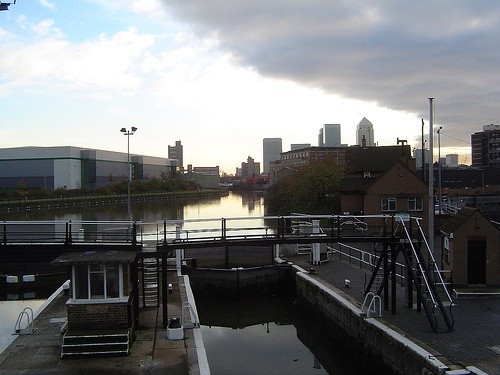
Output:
[120,126,137,237]
